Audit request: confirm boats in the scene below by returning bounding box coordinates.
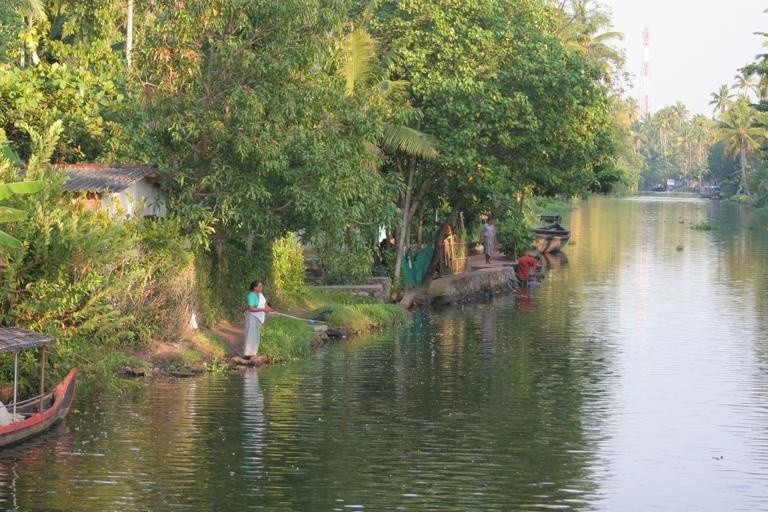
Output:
[541,251,568,273]
[526,220,570,254]
[700,185,721,199]
[0,324,80,450]
[652,183,666,192]
[539,212,562,225]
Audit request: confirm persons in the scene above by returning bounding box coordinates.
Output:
[517,249,539,290]
[477,217,497,264]
[239,366,264,476]
[245,279,274,358]
